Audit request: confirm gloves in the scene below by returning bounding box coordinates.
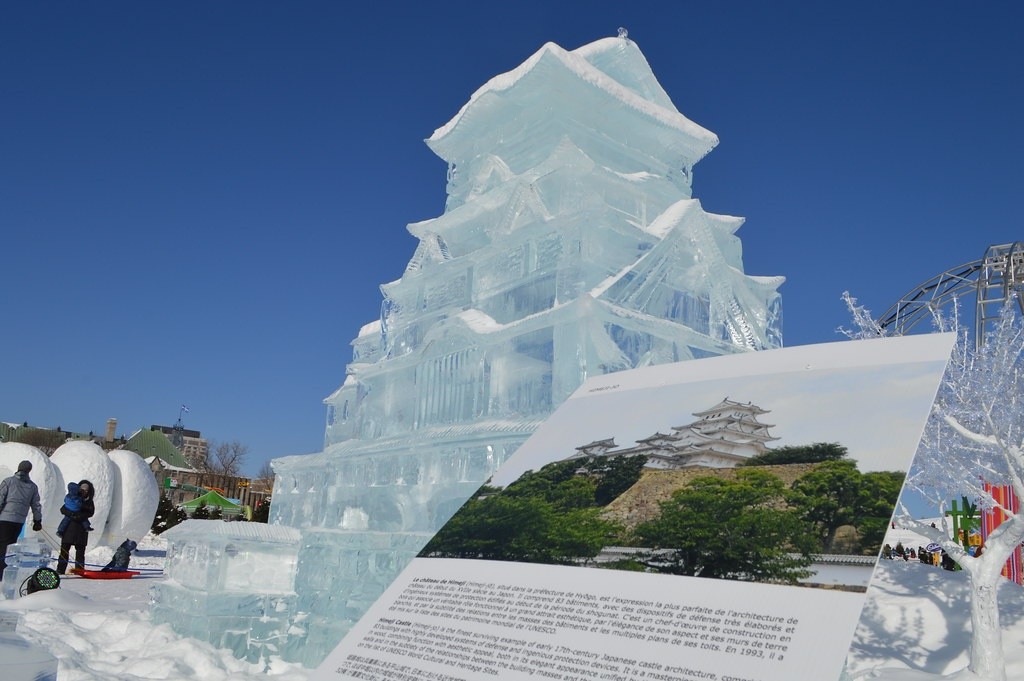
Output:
[32,519,42,531]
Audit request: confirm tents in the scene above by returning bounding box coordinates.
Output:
[176,489,241,522]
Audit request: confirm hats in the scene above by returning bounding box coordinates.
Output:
[126,538,139,552]
[18,461,32,473]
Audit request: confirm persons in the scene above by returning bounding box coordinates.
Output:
[101,538,137,571]
[56,482,95,538]
[0,460,44,581]
[883,541,954,571]
[55,480,95,575]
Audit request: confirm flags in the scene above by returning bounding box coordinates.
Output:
[182,404,189,412]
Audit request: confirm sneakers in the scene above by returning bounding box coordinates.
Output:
[56,530,64,538]
[55,570,65,574]
[85,526,94,531]
